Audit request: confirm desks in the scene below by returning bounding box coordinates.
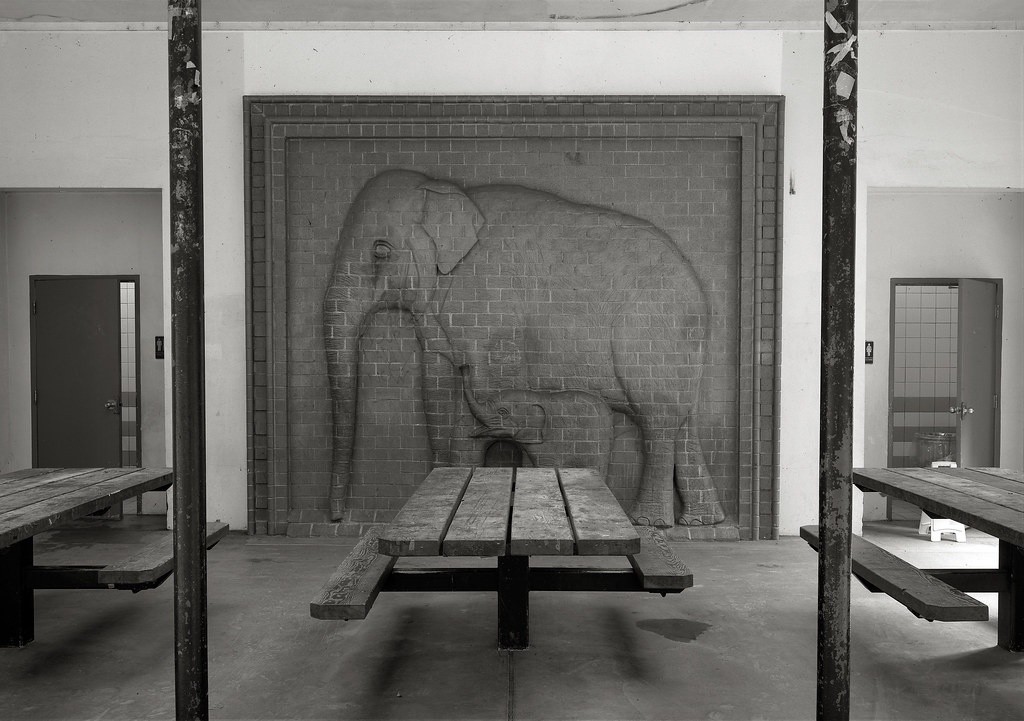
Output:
[853,469,1024,653]
[0,469,176,649]
[378,469,640,653]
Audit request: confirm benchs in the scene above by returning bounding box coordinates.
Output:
[626,526,694,590]
[98,522,229,585]
[310,528,400,621]
[799,525,990,623]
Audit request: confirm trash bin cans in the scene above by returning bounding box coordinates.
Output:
[913,432,956,468]
